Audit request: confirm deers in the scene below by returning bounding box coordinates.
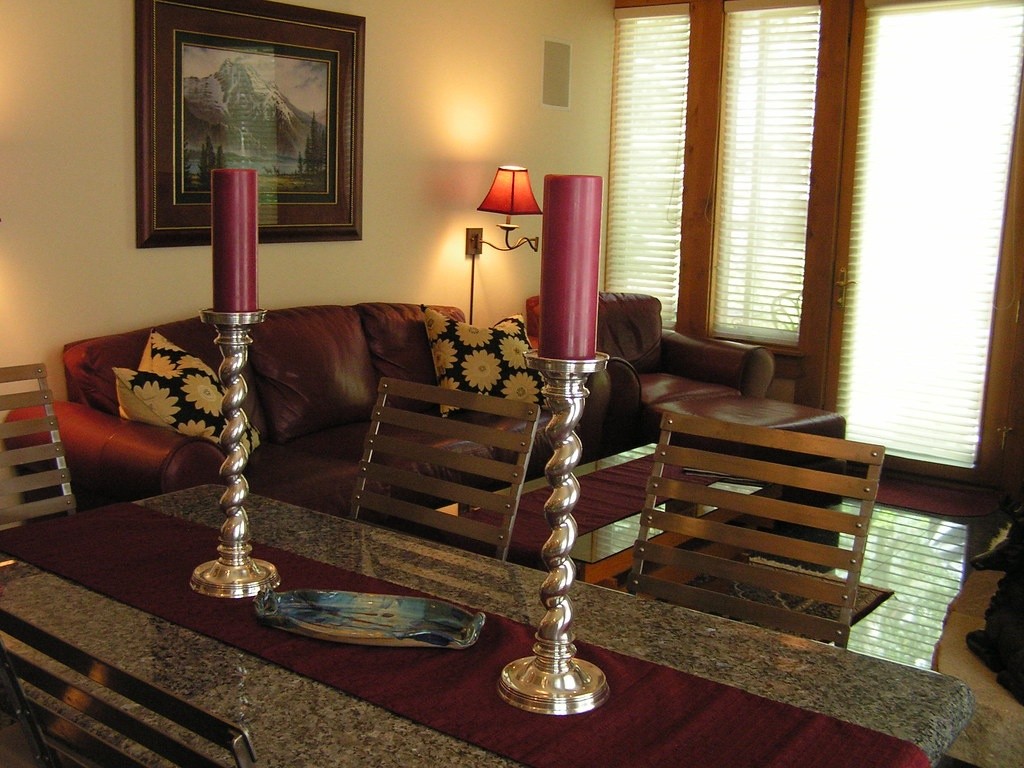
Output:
[965,492,1024,708]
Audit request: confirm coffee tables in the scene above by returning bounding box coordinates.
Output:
[436,443,780,585]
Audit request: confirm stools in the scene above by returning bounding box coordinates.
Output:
[657,394,846,516]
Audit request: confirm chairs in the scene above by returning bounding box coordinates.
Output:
[1,604,256,768]
[358,377,539,561]
[629,411,885,646]
[0,363,77,528]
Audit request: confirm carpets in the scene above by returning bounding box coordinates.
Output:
[598,551,893,645]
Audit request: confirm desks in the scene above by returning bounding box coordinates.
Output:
[0,475,974,768]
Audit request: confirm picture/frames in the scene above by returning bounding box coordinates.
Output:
[132,0,365,246]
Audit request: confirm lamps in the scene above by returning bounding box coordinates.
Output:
[463,165,541,258]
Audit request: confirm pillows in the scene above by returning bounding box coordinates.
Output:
[110,327,259,452]
[417,302,545,417]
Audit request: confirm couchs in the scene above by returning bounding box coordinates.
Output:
[516,291,776,406]
[6,298,641,507]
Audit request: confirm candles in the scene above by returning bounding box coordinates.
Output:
[213,164,259,308]
[536,176,602,355]
